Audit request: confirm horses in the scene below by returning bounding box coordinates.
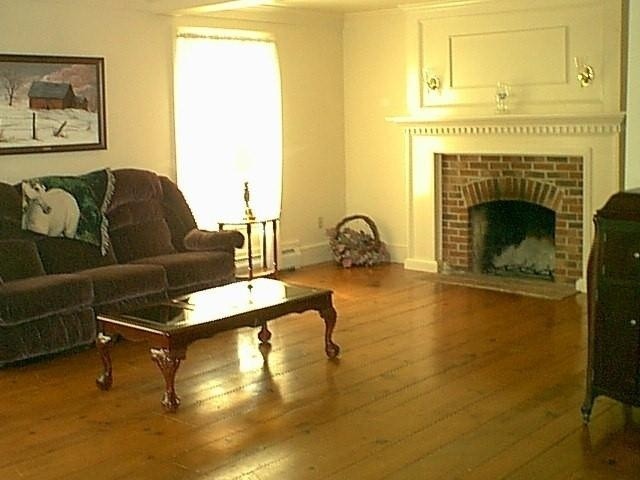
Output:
[22,178,80,239]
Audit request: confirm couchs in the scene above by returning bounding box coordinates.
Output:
[0,169,245,368]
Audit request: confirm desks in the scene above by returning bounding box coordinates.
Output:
[218,213,280,282]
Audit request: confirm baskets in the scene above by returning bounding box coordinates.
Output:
[328,214,383,268]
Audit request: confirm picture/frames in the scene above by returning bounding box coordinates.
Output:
[0,53,110,155]
